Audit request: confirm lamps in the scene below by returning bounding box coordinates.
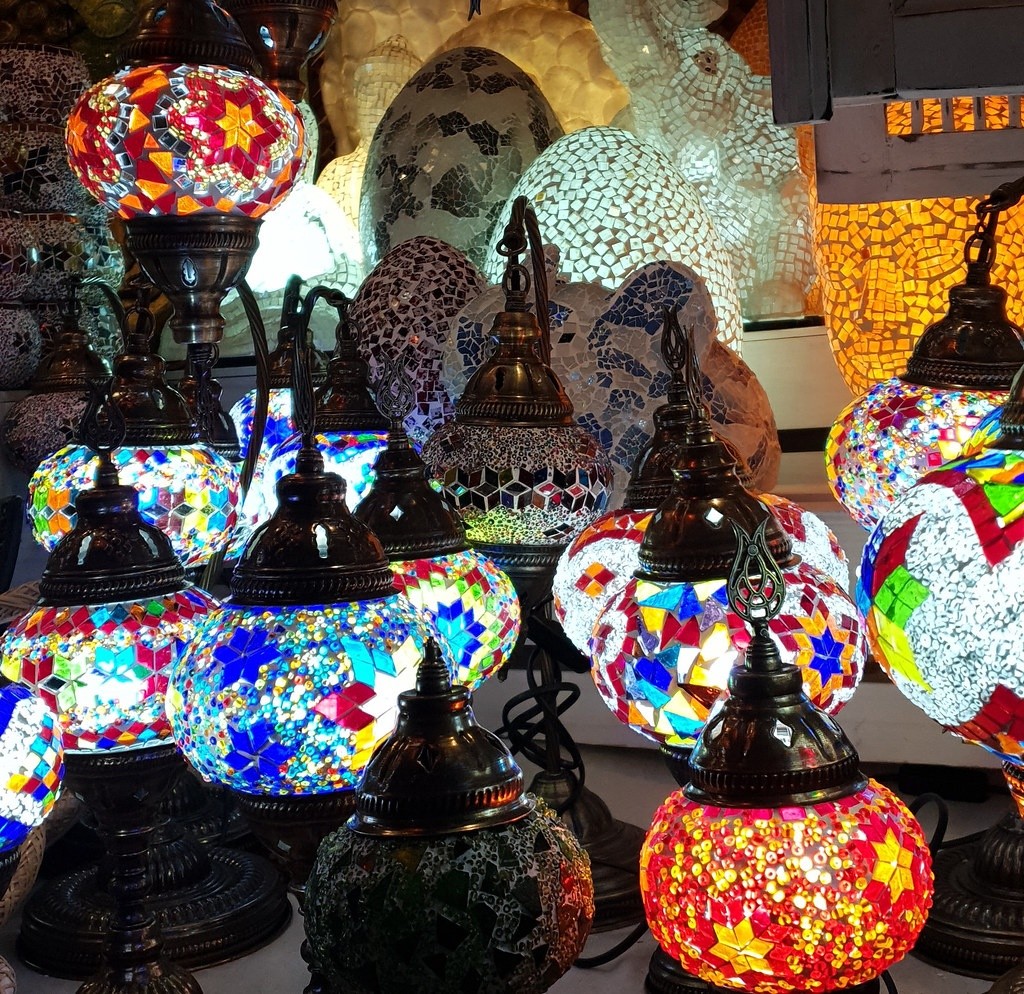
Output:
[0,341,291,983]
[1,681,68,994]
[22,304,244,585]
[589,324,869,790]
[637,515,936,994]
[259,314,393,517]
[548,296,850,664]
[355,348,520,693]
[855,362,1023,983]
[421,194,615,681]
[63,1,310,346]
[298,636,596,993]
[229,272,330,525]
[825,174,1024,531]
[162,282,460,919]
